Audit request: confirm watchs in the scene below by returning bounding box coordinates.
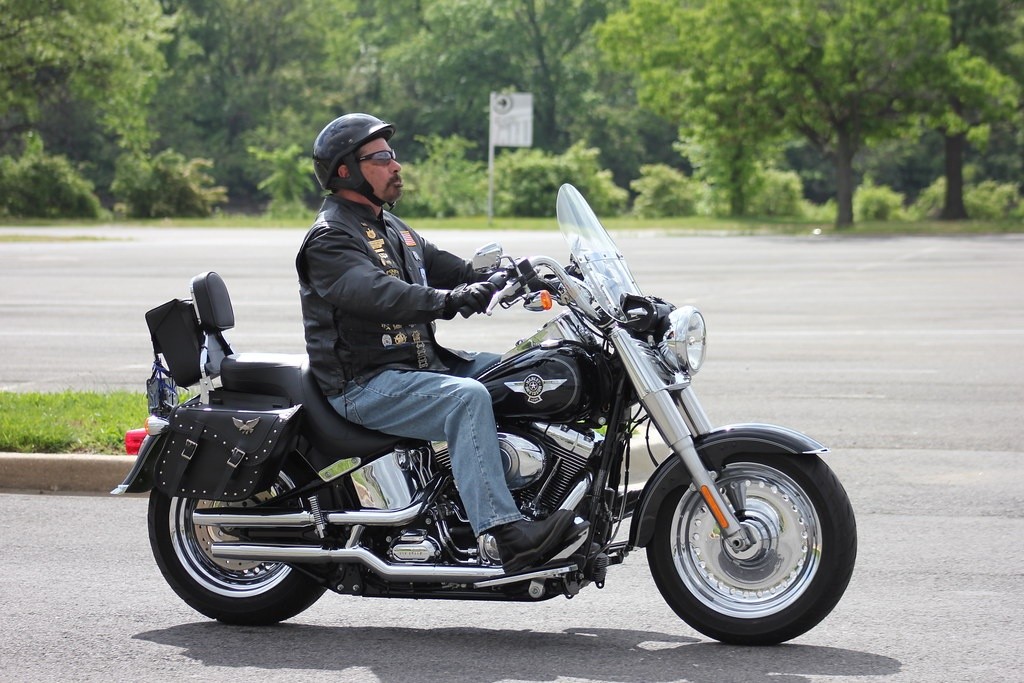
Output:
[450,282,469,306]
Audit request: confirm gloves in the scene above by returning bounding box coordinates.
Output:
[449,281,499,314]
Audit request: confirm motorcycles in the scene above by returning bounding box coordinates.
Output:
[110,182,859,647]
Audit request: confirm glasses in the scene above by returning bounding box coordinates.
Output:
[357,148,397,163]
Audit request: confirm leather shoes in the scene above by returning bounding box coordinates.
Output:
[492,508,577,575]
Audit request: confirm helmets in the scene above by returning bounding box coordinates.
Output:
[312,113,396,191]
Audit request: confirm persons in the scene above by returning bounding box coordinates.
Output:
[294,112,574,574]
